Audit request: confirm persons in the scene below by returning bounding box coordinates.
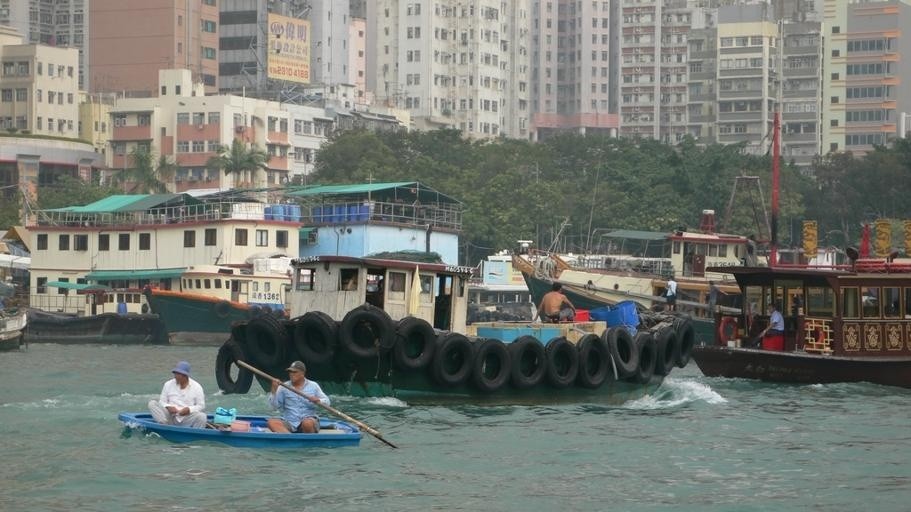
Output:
[148,361,206,428]
[534,282,576,322]
[267,361,331,433]
[708,280,728,317]
[751,304,784,345]
[667,275,677,310]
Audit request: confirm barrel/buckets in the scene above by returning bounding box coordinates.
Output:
[798,307,804,315]
[118,302,127,312]
[727,340,736,346]
[574,309,590,320]
[213,407,238,425]
[232,420,250,431]
[736,339,741,347]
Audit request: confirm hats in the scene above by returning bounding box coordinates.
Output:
[284,360,308,373]
[172,361,193,377]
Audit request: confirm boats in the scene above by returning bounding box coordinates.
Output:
[140,271,293,346]
[509,210,911,388]
[23,308,172,346]
[0,330,24,352]
[214,250,698,397]
[116,412,364,449]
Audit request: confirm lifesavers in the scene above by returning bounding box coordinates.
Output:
[797,322,824,354]
[720,316,738,345]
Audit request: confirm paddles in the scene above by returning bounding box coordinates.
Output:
[236,360,398,448]
